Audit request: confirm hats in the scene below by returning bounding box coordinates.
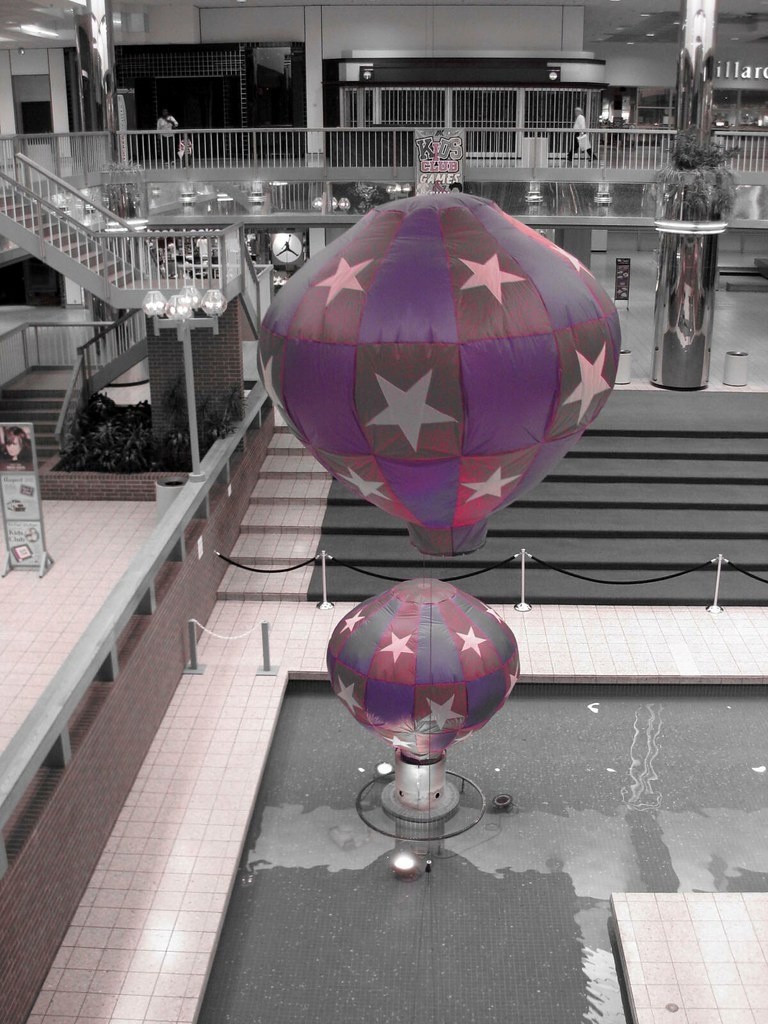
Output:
[576,107,582,112]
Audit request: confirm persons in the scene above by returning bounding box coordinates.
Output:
[156,109,179,165]
[567,107,598,163]
[0,426,32,463]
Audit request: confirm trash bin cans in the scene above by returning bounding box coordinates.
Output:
[711,137,724,154]
[155,477,186,523]
[722,350,749,387]
[522,138,548,168]
[614,350,632,384]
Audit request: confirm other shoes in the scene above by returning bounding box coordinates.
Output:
[589,157,597,161]
[164,162,168,165]
[183,167,188,169]
[171,160,174,165]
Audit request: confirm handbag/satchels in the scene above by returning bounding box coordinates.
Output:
[577,134,591,151]
